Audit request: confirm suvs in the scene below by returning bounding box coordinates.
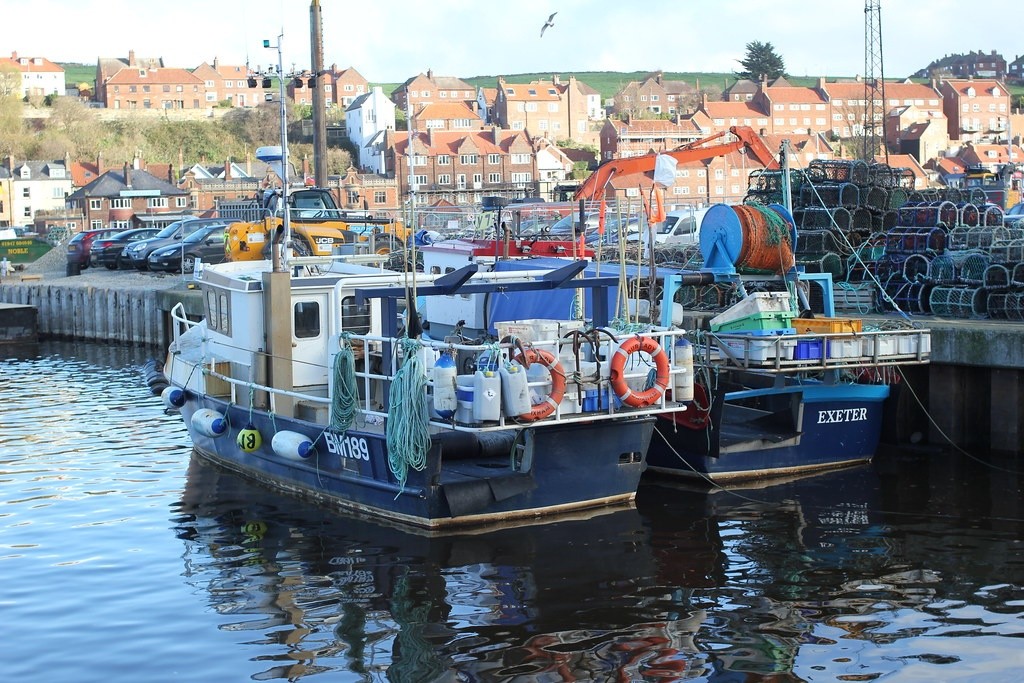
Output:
[120,218,246,271]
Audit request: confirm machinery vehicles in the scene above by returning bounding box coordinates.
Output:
[501,124,781,221]
[224,182,412,269]
[959,166,1024,215]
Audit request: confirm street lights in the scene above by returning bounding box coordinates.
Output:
[987,108,1013,166]
[404,81,434,271]
[246,32,294,266]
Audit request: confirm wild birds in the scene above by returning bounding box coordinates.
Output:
[540,12,558,37]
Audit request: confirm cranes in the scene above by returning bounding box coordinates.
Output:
[864,1,891,170]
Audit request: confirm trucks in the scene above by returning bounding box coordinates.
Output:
[640,207,711,249]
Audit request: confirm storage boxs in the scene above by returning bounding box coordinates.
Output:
[428,289,932,426]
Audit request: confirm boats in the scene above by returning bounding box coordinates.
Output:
[143,237,689,535]
[420,135,931,490]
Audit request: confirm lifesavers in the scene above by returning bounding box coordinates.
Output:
[516,350,565,420]
[612,336,670,408]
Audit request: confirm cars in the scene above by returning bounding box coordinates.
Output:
[90,228,162,270]
[147,224,231,274]
[67,227,128,270]
[446,209,647,251]
